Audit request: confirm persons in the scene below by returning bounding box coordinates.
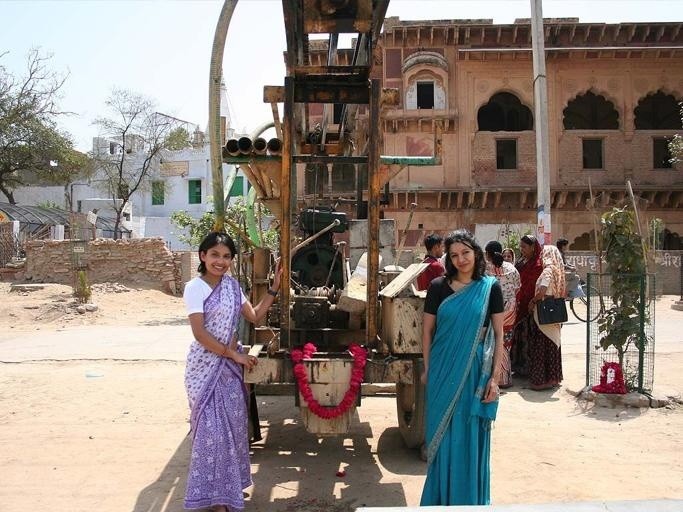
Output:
[416,235,585,391]
[420,230,506,505]
[183,232,281,511]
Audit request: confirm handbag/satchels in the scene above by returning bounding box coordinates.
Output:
[537,295,568,324]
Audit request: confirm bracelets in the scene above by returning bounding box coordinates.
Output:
[267,287,279,297]
[220,345,228,358]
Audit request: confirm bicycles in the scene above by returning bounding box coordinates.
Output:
[564,265,603,323]
